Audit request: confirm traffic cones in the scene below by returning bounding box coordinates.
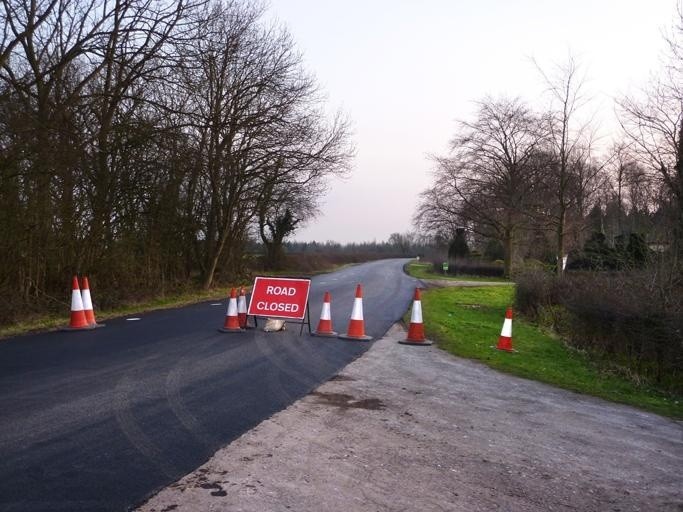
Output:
[490,303,521,353]
[311,291,337,337]
[82,276,106,330]
[398,286,434,347]
[219,287,246,334]
[239,286,255,329]
[338,284,373,341]
[59,274,90,330]
